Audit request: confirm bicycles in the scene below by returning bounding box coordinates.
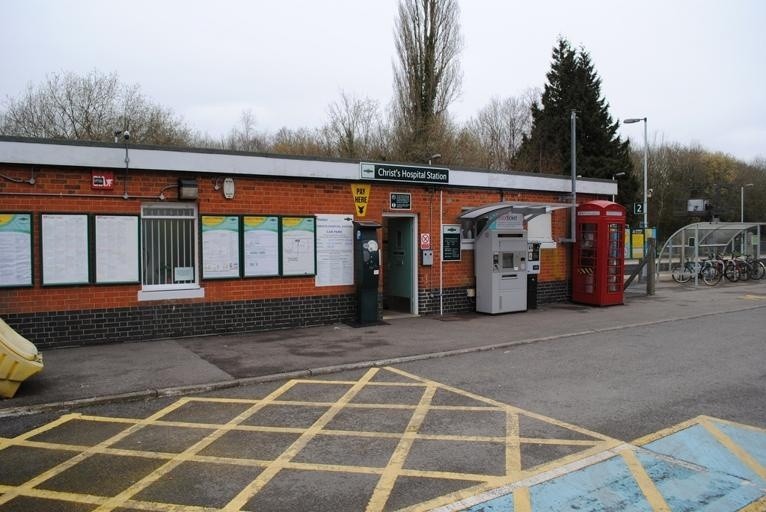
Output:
[671,246,765,285]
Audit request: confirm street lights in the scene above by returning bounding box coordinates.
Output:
[612,171,626,202]
[428,155,442,166]
[740,183,753,222]
[624,116,648,266]
